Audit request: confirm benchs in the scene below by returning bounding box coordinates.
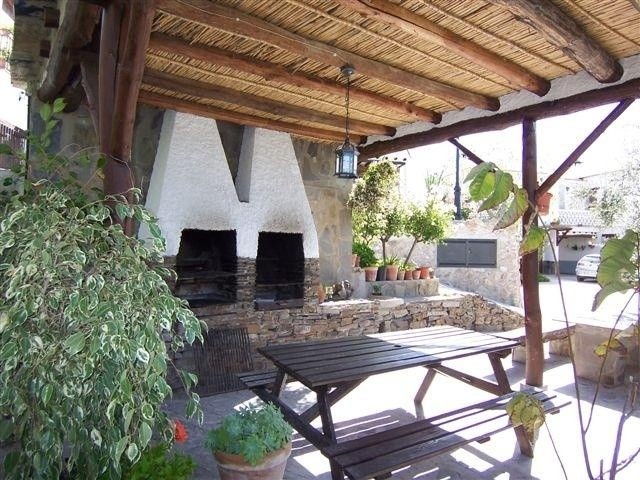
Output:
[233,363,298,398]
[498,312,574,363]
[317,383,572,480]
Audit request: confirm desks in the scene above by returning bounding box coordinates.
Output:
[255,320,537,480]
[549,308,639,389]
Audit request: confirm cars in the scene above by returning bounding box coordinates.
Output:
[575,253,600,282]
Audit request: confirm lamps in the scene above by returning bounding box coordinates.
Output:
[331,59,361,180]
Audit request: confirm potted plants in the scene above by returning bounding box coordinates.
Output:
[351,241,435,283]
[207,395,296,480]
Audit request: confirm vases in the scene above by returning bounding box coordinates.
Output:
[535,190,554,216]
